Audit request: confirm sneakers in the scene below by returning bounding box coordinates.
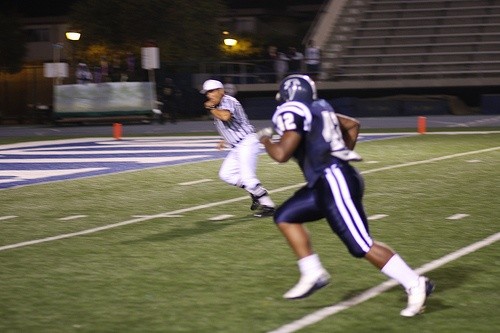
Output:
[250,194,260,210]
[399,277,433,316]
[254,205,275,216]
[282,268,332,298]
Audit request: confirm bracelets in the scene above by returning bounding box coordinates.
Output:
[210,105,217,109]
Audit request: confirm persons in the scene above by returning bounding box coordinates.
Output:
[200,79,277,218]
[74,54,136,84]
[261,40,322,84]
[259,74,436,317]
[158,76,181,125]
[223,77,237,96]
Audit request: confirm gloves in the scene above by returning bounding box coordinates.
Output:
[255,126,273,140]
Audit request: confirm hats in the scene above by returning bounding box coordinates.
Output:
[200,79,224,94]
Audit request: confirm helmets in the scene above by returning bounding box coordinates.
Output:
[279,74,317,102]
